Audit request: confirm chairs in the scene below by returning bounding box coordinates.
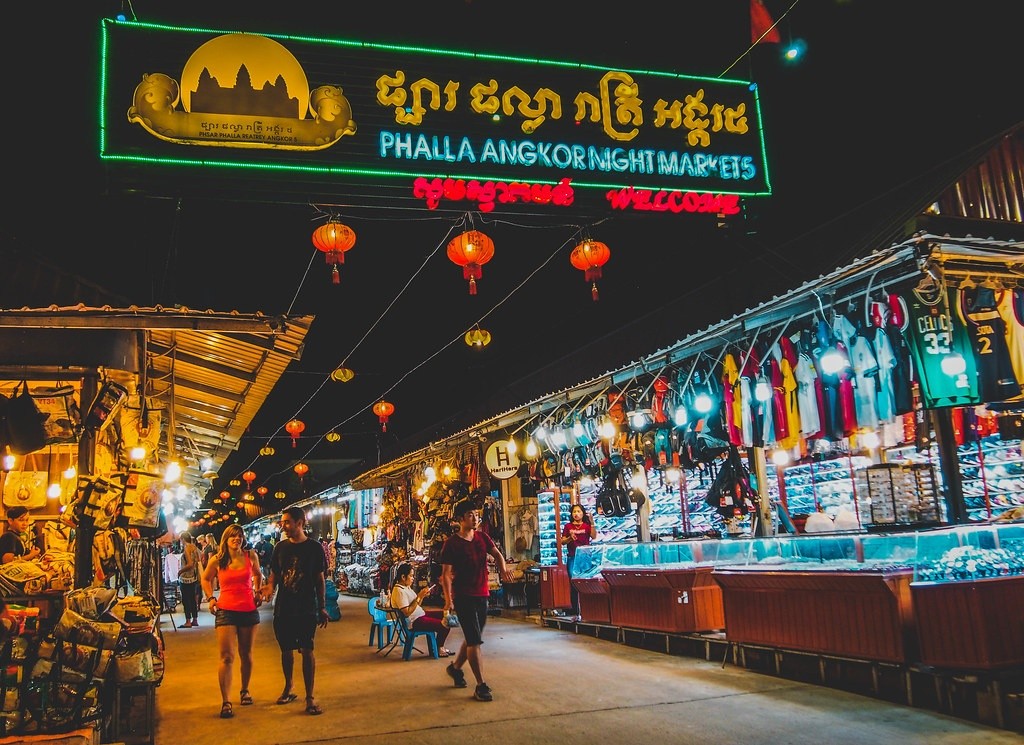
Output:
[395,608,439,661]
[367,597,404,649]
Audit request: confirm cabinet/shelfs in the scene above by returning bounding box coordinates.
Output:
[537,438,1024,608]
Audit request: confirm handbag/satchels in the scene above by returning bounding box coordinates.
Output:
[86,366,128,432]
[596,470,645,517]
[120,398,164,457]
[56,608,123,649]
[0,520,128,596]
[0,381,48,454]
[38,634,116,678]
[706,445,756,519]
[23,680,102,734]
[30,384,80,445]
[118,468,163,529]
[4,455,49,509]
[114,647,155,682]
[61,475,124,531]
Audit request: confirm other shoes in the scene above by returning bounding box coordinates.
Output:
[439,650,455,658]
[30,657,105,684]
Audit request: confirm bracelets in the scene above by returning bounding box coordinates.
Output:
[590,524,595,528]
[207,597,217,602]
[415,596,422,603]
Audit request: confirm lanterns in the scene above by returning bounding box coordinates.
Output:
[312,221,356,284]
[570,237,610,302]
[190,471,265,527]
[293,462,308,479]
[447,229,494,294]
[286,419,304,448]
[374,400,394,433]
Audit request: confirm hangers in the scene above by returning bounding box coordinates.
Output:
[796,268,1020,355]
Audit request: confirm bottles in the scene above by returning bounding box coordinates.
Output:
[379,589,385,607]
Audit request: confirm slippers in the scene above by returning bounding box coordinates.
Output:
[306,704,322,715]
[177,625,192,628]
[277,693,297,705]
[191,622,198,626]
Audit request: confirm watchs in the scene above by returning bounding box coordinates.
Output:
[253,589,262,594]
[319,606,325,611]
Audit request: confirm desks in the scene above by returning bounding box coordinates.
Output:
[374,605,425,657]
[112,682,155,745]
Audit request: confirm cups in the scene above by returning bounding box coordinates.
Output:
[384,595,390,607]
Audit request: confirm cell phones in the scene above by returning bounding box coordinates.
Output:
[429,584,436,590]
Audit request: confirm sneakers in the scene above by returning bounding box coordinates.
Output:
[447,660,468,687]
[475,682,493,701]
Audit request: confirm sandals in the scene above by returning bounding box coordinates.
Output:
[220,701,233,718]
[240,690,252,705]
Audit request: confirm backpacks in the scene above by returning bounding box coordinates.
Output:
[524,366,715,482]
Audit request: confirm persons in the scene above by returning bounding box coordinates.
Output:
[440,499,507,701]
[192,532,220,603]
[391,562,455,657]
[201,524,262,718]
[241,524,281,602]
[256,503,331,714]
[177,532,201,628]
[561,504,597,622]
[0,505,40,564]
